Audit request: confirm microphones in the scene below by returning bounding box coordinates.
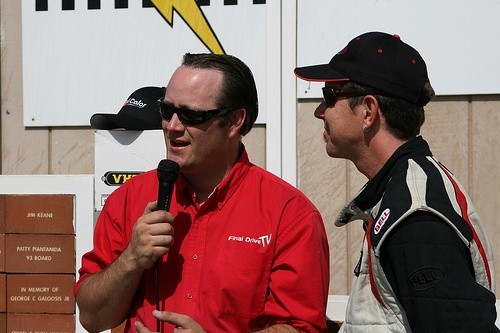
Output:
[153,159,181,267]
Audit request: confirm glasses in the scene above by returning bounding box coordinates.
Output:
[321,87,394,108]
[156,97,240,126]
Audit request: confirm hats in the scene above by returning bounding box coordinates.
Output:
[90,87,165,130]
[295,32,430,107]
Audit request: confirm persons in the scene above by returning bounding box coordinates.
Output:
[294,33,500,333]
[73,53,330,333]
